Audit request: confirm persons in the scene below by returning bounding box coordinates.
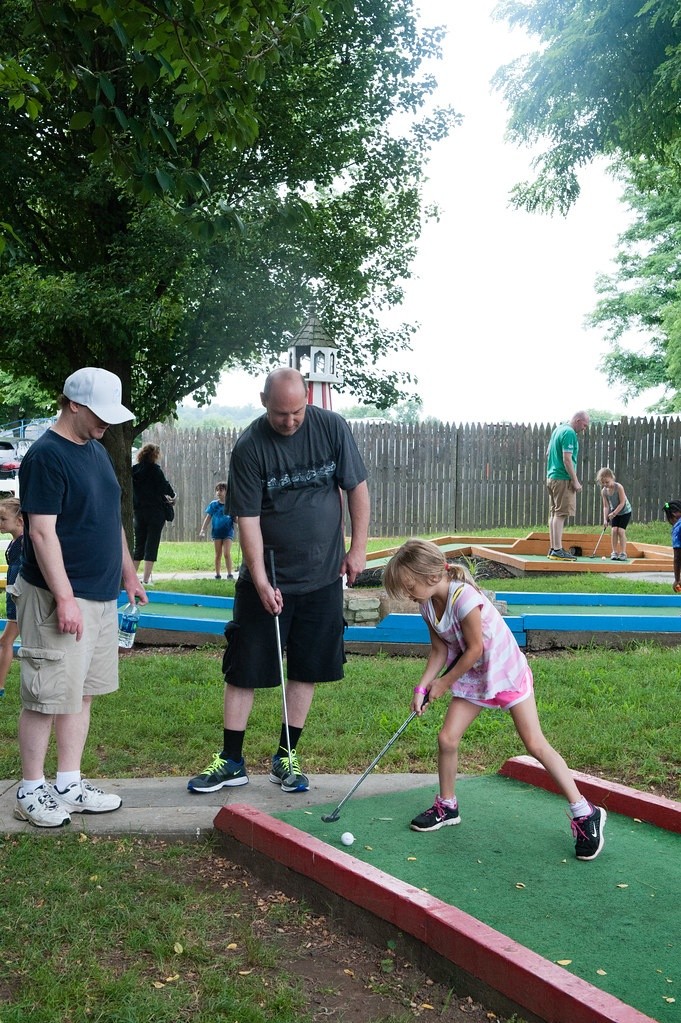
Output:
[546,409,589,560]
[597,467,633,561]
[0,499,25,700]
[199,481,238,580]
[12,367,148,828]
[188,367,370,802]
[132,443,178,587]
[662,500,681,593]
[373,537,607,861]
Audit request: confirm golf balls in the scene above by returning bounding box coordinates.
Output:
[342,832,354,846]
[602,556,606,561]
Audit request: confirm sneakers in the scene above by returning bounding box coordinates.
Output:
[52,778,123,814]
[187,751,249,792]
[610,552,618,559]
[565,803,607,860]
[409,794,461,832]
[14,782,72,829]
[547,548,578,561]
[617,553,627,561]
[269,746,310,792]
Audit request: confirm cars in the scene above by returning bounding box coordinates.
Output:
[0,436,36,498]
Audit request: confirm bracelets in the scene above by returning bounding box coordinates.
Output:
[414,686,426,695]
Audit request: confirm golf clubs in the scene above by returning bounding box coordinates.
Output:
[320,648,463,824]
[270,549,297,785]
[235,541,240,572]
[589,508,614,558]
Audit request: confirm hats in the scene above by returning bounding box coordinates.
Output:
[63,367,136,425]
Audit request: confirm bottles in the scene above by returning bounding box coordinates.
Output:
[118,597,140,648]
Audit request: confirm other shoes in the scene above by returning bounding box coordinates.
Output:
[140,580,155,586]
[227,574,234,579]
[215,575,221,581]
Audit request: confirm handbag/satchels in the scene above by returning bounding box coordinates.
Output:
[165,497,174,522]
